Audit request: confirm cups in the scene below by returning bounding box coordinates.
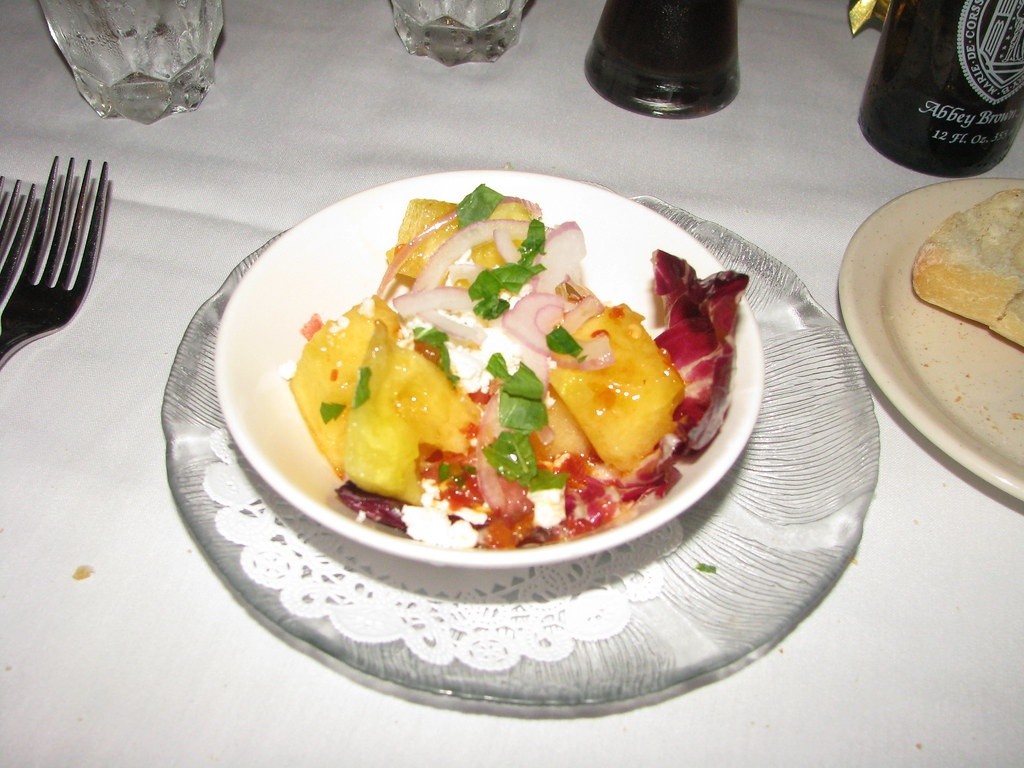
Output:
[391,0,528,65]
[36,0,228,124]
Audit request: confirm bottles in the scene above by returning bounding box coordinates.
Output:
[853,0,1024,177]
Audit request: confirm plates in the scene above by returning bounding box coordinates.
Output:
[213,170,763,572]
[838,179,1024,504]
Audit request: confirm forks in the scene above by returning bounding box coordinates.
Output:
[0,153,108,368]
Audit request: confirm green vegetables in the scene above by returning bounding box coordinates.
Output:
[320,182,582,491]
